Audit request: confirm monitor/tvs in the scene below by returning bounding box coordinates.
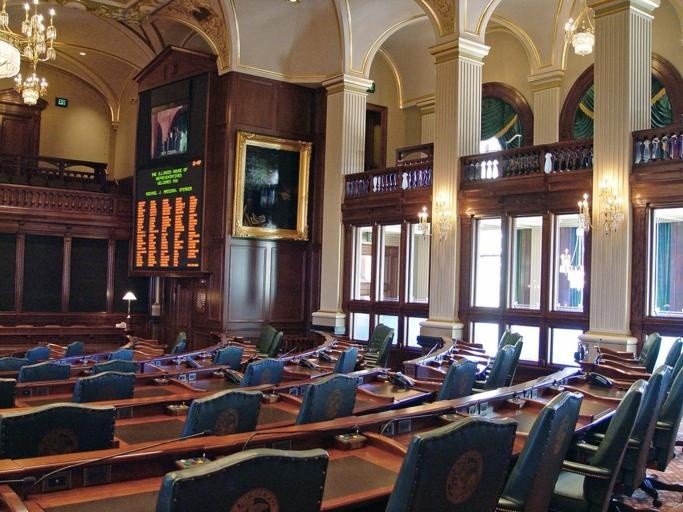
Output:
[147,97,190,161]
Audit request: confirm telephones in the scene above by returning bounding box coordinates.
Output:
[186,359,201,369]
[299,359,316,370]
[224,369,245,384]
[584,372,613,388]
[319,352,331,362]
[390,373,415,388]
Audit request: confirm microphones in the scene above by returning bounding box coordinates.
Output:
[242,425,360,451]
[0,476,36,484]
[425,342,439,358]
[598,339,602,359]
[261,384,279,390]
[509,364,520,390]
[24,429,212,495]
[380,411,454,434]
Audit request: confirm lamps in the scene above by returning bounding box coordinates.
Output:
[576,181,628,237]
[0,0,60,109]
[121,291,138,322]
[563,2,600,56]
[413,201,455,244]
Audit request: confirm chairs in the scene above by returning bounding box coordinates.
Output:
[0,322,683,512]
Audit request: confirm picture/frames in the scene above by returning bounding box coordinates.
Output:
[232,130,312,241]
